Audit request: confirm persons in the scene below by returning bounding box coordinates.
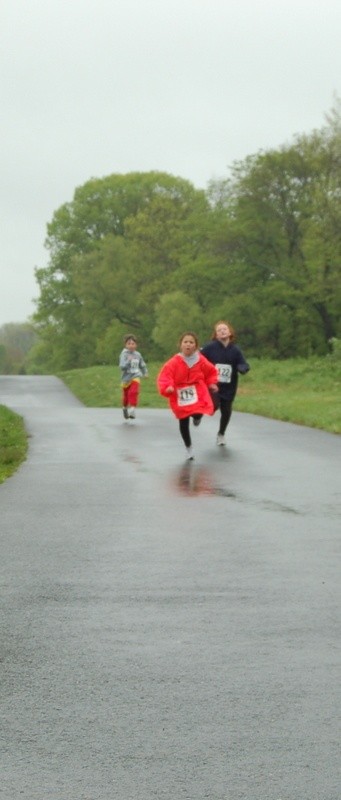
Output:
[200,320,250,446]
[157,332,220,460]
[119,335,147,419]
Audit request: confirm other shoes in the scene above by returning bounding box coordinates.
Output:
[216,431,226,445]
[193,418,201,426]
[123,406,135,419]
[186,446,194,460]
